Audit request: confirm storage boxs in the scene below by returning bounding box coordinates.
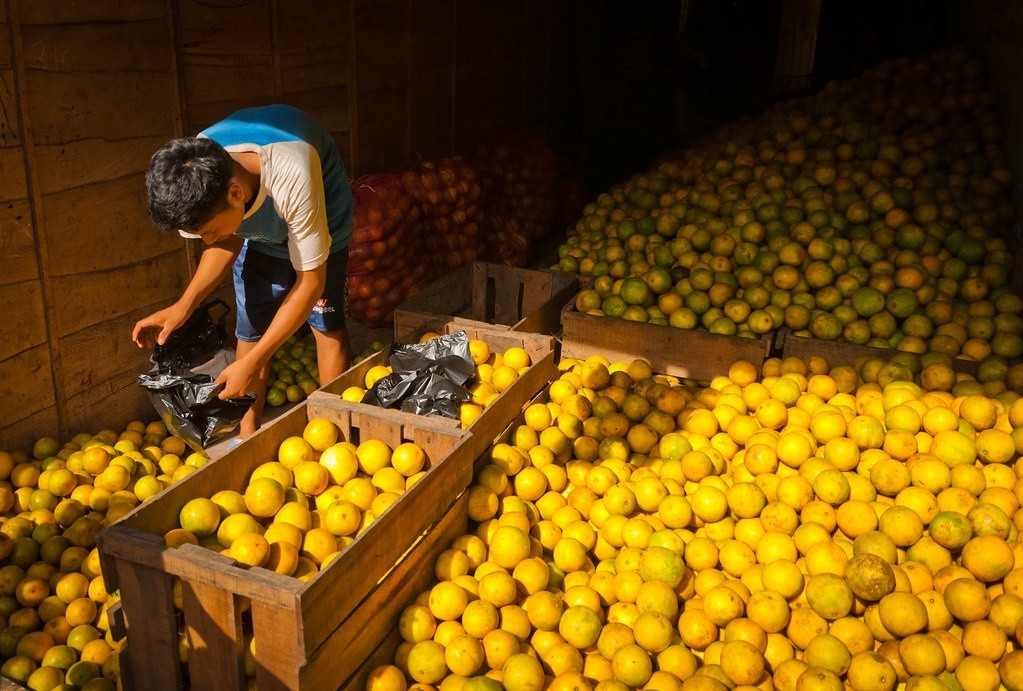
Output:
[94,221,1022,691]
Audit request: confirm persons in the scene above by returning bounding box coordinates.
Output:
[132,104,352,449]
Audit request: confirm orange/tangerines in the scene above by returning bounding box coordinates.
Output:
[0,99,1023,691]
